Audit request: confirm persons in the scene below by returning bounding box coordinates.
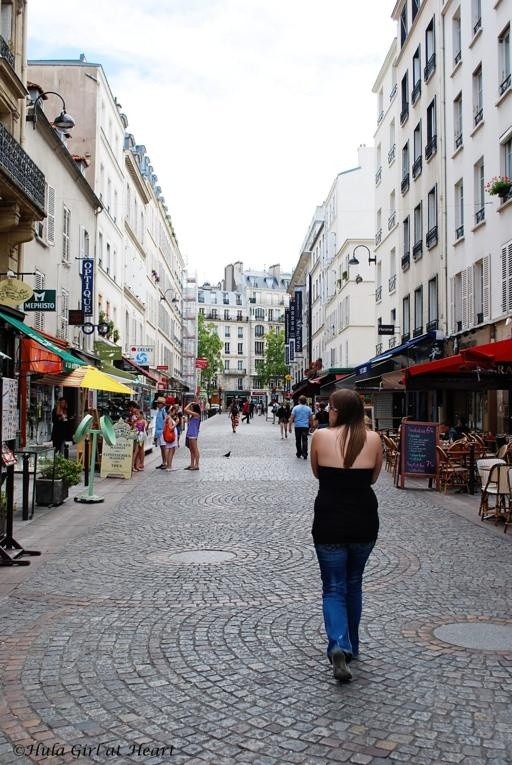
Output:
[51,397,71,454]
[125,396,202,473]
[310,388,384,682]
[227,395,329,459]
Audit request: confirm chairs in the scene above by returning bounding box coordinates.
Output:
[377,421,512,536]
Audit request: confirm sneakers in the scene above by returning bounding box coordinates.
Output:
[332,649,353,681]
[133,466,144,472]
[156,464,171,469]
[184,465,199,470]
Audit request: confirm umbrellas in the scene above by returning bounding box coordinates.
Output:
[31,364,139,418]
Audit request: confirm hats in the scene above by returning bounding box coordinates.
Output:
[154,396,166,404]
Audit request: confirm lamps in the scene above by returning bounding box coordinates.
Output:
[347,243,377,268]
[24,88,74,131]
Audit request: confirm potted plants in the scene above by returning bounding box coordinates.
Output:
[33,452,84,508]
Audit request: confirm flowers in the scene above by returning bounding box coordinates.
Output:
[482,172,512,205]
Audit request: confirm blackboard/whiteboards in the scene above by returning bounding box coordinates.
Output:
[401,420,440,478]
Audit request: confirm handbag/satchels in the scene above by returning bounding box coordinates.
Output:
[163,418,175,442]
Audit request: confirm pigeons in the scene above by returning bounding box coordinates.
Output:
[223,451,231,457]
